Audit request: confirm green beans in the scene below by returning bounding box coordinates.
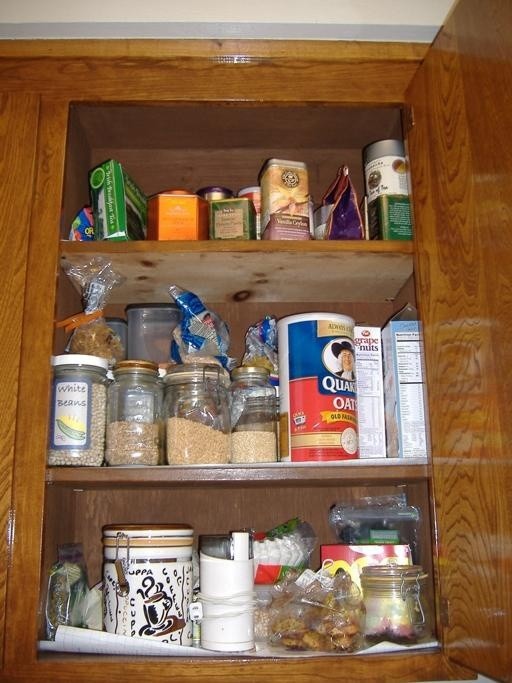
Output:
[47,383,107,466]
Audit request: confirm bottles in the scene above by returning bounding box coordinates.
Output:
[199,533,257,651]
[48,352,279,467]
[359,564,434,648]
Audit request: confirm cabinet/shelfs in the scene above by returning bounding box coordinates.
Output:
[1,0,512,683]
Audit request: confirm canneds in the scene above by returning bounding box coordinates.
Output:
[361,137,409,204]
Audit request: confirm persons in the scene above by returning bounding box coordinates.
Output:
[331,341,357,382]
